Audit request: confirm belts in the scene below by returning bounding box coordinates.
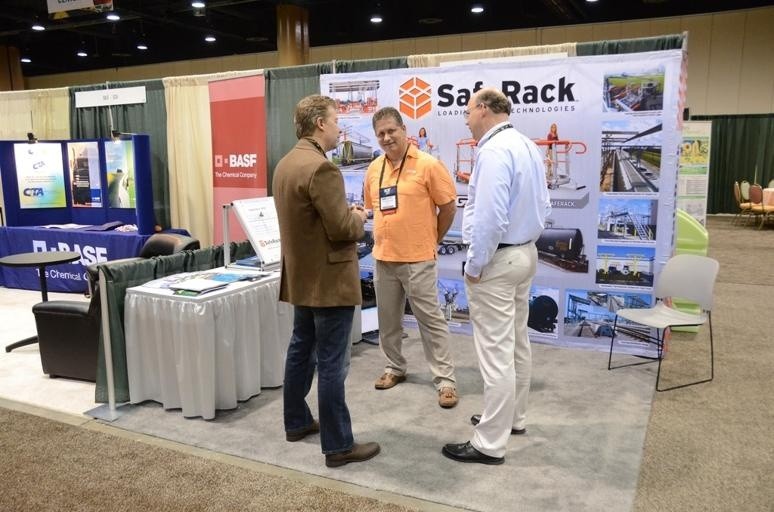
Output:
[497,240,531,249]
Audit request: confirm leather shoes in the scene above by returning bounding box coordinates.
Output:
[471,415,525,434]
[326,442,379,467]
[442,440,504,465]
[286,420,320,442]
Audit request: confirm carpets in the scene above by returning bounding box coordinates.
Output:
[86,311,659,512]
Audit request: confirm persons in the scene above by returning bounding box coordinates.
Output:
[361,107,458,408]
[443,288,458,320]
[544,123,559,179]
[443,86,552,465]
[269,94,380,468]
[416,127,433,152]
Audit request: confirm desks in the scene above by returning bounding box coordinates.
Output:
[0,251,81,352]
[0,223,192,294]
[125,257,362,420]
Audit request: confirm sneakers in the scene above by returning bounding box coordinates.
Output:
[437,388,458,407]
[375,373,405,389]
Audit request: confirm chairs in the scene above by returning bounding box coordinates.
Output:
[33,257,148,383]
[86,232,200,301]
[608,254,719,392]
[734,179,774,230]
